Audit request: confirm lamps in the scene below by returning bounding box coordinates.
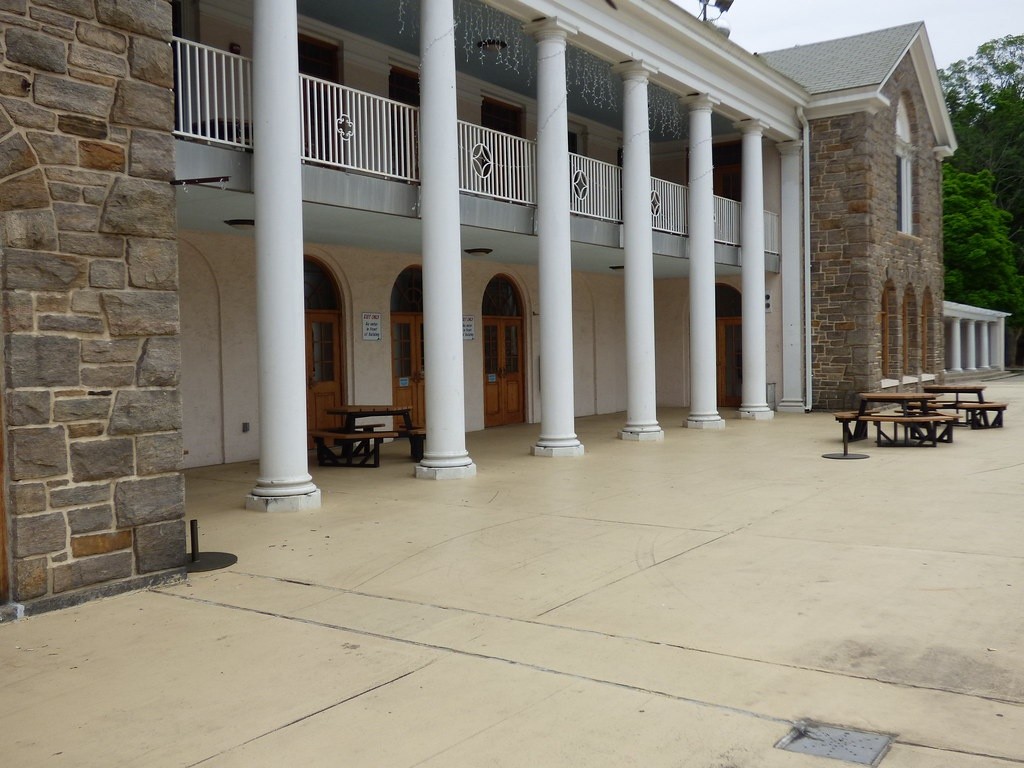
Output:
[478,39,506,53]
[464,247,493,256]
[610,265,625,271]
[223,219,255,230]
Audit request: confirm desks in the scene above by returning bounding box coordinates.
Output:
[327,405,413,459]
[922,385,989,426]
[853,393,936,441]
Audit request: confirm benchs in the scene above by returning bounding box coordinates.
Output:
[822,400,1008,460]
[308,424,426,470]
